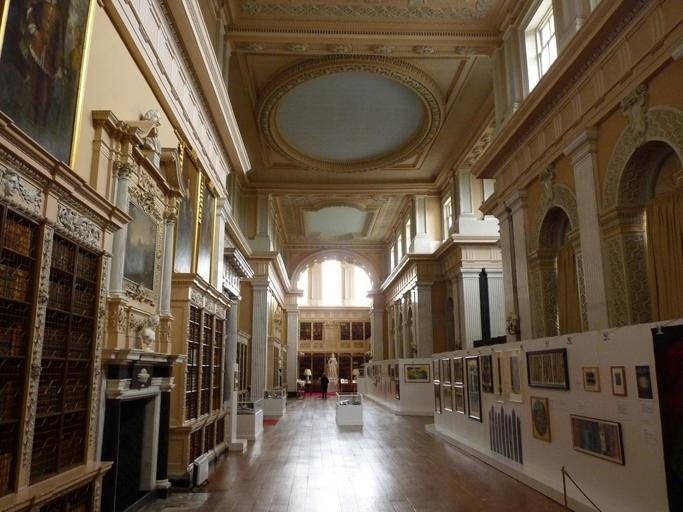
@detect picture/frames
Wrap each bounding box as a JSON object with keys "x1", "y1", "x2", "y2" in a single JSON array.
[{"x1": 373, "y1": 348, "x2": 653, "y2": 465}]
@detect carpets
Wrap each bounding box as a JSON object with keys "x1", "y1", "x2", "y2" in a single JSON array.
[{"x1": 263, "y1": 418, "x2": 279, "y2": 426}]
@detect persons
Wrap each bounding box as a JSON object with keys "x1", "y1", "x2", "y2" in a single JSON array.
[
  {"x1": 22, "y1": 0, "x2": 66, "y2": 131},
  {"x1": 319, "y1": 373, "x2": 329, "y2": 399},
  {"x1": 328, "y1": 352, "x2": 338, "y2": 379}
]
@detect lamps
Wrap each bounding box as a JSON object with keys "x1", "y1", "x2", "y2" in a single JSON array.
[
  {"x1": 352, "y1": 369, "x2": 361, "y2": 383},
  {"x1": 303, "y1": 369, "x2": 312, "y2": 384}
]
